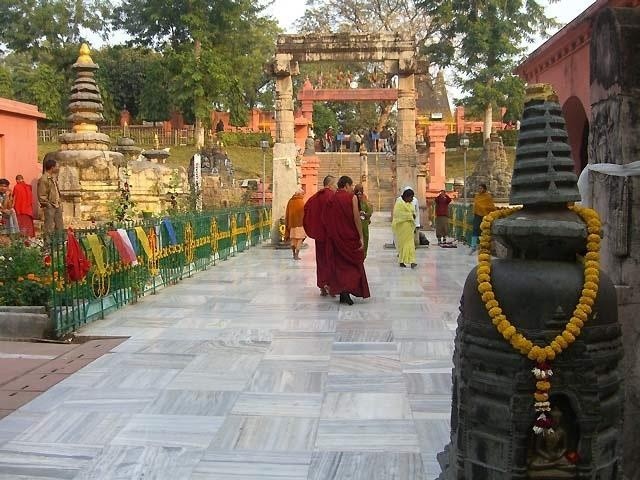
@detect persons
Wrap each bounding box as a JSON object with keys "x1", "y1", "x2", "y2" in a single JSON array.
[
  {"x1": 351, "y1": 184, "x2": 374, "y2": 261},
  {"x1": 529, "y1": 405, "x2": 577, "y2": 472},
  {"x1": 433, "y1": 190, "x2": 452, "y2": 243},
  {"x1": 396, "y1": 186, "x2": 420, "y2": 257},
  {"x1": 0, "y1": 179, "x2": 15, "y2": 237},
  {"x1": 391, "y1": 189, "x2": 418, "y2": 268},
  {"x1": 36, "y1": 158, "x2": 63, "y2": 234},
  {"x1": 468, "y1": 183, "x2": 496, "y2": 256},
  {"x1": 12, "y1": 175, "x2": 35, "y2": 238},
  {"x1": 320, "y1": 175, "x2": 371, "y2": 305},
  {"x1": 286, "y1": 187, "x2": 308, "y2": 260},
  {"x1": 304, "y1": 175, "x2": 338, "y2": 297},
  {"x1": 322, "y1": 126, "x2": 392, "y2": 152}
]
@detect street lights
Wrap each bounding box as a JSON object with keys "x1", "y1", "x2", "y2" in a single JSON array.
[
  {"x1": 257, "y1": 135, "x2": 270, "y2": 241},
  {"x1": 458, "y1": 130, "x2": 471, "y2": 244}
]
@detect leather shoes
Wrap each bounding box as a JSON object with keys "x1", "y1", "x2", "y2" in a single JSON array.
[
  {"x1": 400, "y1": 263, "x2": 407, "y2": 267},
  {"x1": 411, "y1": 263, "x2": 417, "y2": 269}
]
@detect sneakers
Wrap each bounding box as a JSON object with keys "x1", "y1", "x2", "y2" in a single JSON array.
[
  {"x1": 320, "y1": 284, "x2": 329, "y2": 296},
  {"x1": 339, "y1": 293, "x2": 354, "y2": 306}
]
